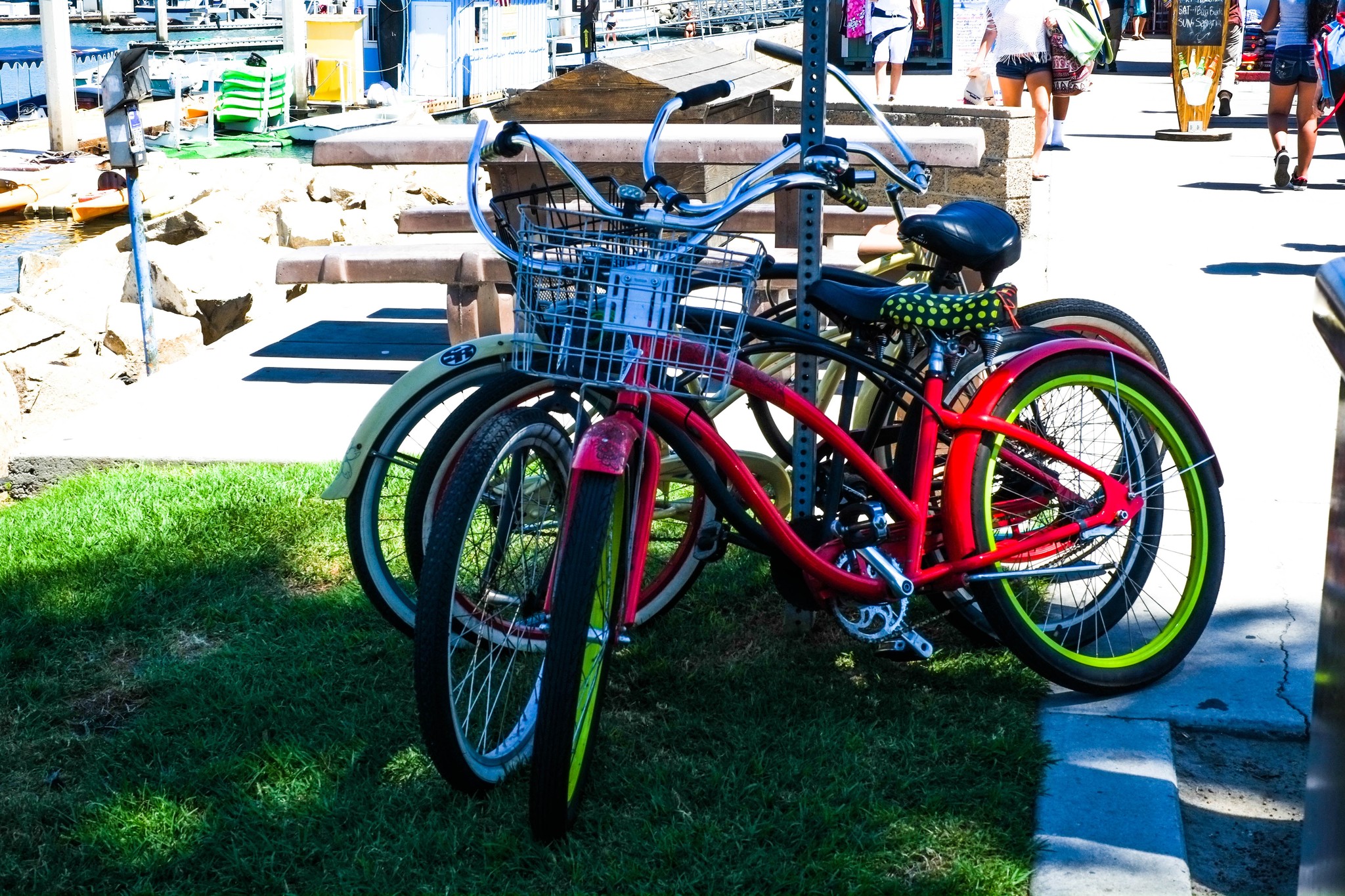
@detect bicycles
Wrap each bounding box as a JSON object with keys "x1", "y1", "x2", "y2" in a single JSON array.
[{"x1": 317, "y1": 37, "x2": 1226, "y2": 848}]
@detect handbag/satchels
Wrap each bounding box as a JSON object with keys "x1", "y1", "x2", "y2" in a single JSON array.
[
  {"x1": 1050, "y1": 27, "x2": 1093, "y2": 98},
  {"x1": 964, "y1": 74, "x2": 996, "y2": 106}
]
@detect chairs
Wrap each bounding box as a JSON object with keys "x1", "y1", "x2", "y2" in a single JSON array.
[{"x1": 0, "y1": 110, "x2": 10, "y2": 121}]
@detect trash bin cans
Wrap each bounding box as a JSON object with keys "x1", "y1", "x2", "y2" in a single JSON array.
[{"x1": 1293, "y1": 253, "x2": 1345, "y2": 892}]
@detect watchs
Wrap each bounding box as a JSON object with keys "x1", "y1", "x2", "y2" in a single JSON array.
[{"x1": 917, "y1": 12, "x2": 924, "y2": 18}]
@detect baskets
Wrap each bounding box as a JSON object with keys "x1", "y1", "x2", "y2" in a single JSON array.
[
  {"x1": 510, "y1": 204, "x2": 768, "y2": 401},
  {"x1": 490, "y1": 177, "x2": 709, "y2": 354}
]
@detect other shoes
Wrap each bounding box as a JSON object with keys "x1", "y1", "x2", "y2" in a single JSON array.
[
  {"x1": 1030, "y1": 170, "x2": 1045, "y2": 181},
  {"x1": 1139, "y1": 35, "x2": 1144, "y2": 40},
  {"x1": 1132, "y1": 35, "x2": 1141, "y2": 40},
  {"x1": 1218, "y1": 91, "x2": 1232, "y2": 117},
  {"x1": 1097, "y1": 64, "x2": 1105, "y2": 69},
  {"x1": 1108, "y1": 60, "x2": 1117, "y2": 72},
  {"x1": 888, "y1": 94, "x2": 894, "y2": 101}
]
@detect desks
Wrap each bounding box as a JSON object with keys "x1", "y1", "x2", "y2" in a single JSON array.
[
  {"x1": 313, "y1": 121, "x2": 987, "y2": 249},
  {"x1": 40, "y1": 104, "x2": 80, "y2": 107}
]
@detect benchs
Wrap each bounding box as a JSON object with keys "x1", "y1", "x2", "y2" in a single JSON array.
[
  {"x1": 398, "y1": 203, "x2": 941, "y2": 248},
  {"x1": 277, "y1": 249, "x2": 862, "y2": 346}
]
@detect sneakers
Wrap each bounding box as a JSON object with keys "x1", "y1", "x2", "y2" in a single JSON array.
[
  {"x1": 1290, "y1": 173, "x2": 1307, "y2": 190},
  {"x1": 1274, "y1": 145, "x2": 1290, "y2": 188}
]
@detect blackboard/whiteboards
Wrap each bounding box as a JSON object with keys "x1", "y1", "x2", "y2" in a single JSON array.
[{"x1": 1176, "y1": 0, "x2": 1225, "y2": 47}]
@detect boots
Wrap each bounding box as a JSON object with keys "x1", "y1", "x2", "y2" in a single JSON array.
[{"x1": 1052, "y1": 118, "x2": 1064, "y2": 147}]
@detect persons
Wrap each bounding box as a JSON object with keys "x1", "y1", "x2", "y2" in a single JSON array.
[
  {"x1": 871, "y1": 0, "x2": 924, "y2": 103},
  {"x1": 966, "y1": 0, "x2": 1154, "y2": 180},
  {"x1": 1209, "y1": 0, "x2": 1345, "y2": 192}
]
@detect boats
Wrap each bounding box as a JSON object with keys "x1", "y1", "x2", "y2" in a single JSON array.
[
  {"x1": 546, "y1": 0, "x2": 660, "y2": 40},
  {"x1": 0, "y1": 1, "x2": 288, "y2": 227},
  {"x1": 546, "y1": 35, "x2": 598, "y2": 77},
  {"x1": 651, "y1": 0, "x2": 804, "y2": 36}
]
